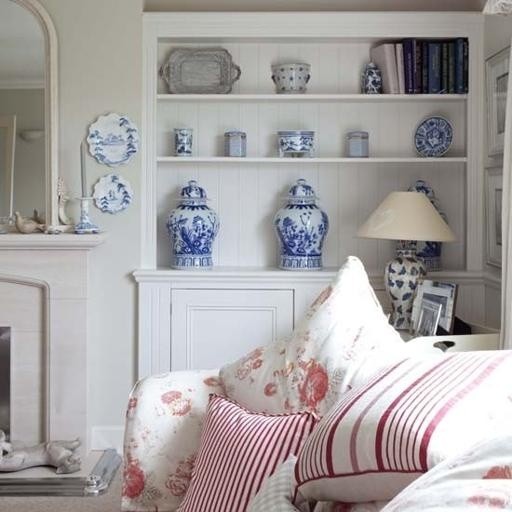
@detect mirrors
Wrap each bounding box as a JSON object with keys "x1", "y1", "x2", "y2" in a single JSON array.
[{"x1": 0, "y1": 0, "x2": 64, "y2": 233}]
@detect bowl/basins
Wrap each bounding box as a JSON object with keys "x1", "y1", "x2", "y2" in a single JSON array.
[{"x1": 277, "y1": 130, "x2": 314, "y2": 154}]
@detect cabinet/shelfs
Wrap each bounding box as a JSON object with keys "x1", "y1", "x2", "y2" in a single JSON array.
[
  {"x1": 133, "y1": 268, "x2": 490, "y2": 378},
  {"x1": 138, "y1": 9, "x2": 484, "y2": 279},
  {"x1": 478, "y1": 275, "x2": 504, "y2": 334}
]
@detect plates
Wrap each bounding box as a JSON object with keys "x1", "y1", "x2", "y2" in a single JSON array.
[
  {"x1": 93, "y1": 173, "x2": 134, "y2": 213},
  {"x1": 40, "y1": 224, "x2": 71, "y2": 234},
  {"x1": 86, "y1": 111, "x2": 138, "y2": 165},
  {"x1": 414, "y1": 116, "x2": 455, "y2": 157},
  {"x1": 164, "y1": 48, "x2": 233, "y2": 94}
]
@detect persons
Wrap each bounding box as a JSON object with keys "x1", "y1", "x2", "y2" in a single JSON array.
[{"x1": 419, "y1": 314, "x2": 434, "y2": 335}]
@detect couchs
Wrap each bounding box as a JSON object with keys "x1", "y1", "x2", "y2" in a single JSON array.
[{"x1": 117, "y1": 342, "x2": 510, "y2": 512}]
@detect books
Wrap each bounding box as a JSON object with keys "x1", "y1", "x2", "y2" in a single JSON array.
[{"x1": 371, "y1": 38, "x2": 468, "y2": 93}]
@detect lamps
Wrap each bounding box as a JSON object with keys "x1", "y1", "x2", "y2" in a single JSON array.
[{"x1": 351, "y1": 189, "x2": 460, "y2": 334}]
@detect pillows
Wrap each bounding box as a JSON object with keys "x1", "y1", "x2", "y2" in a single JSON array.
[
  {"x1": 292, "y1": 348, "x2": 511, "y2": 507},
  {"x1": 174, "y1": 393, "x2": 316, "y2": 512},
  {"x1": 214, "y1": 255, "x2": 404, "y2": 425},
  {"x1": 239, "y1": 454, "x2": 300, "y2": 512},
  {"x1": 382, "y1": 444, "x2": 510, "y2": 512}
]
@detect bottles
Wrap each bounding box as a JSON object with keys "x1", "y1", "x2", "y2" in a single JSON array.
[
  {"x1": 276, "y1": 178, "x2": 331, "y2": 272},
  {"x1": 361, "y1": 59, "x2": 383, "y2": 94},
  {"x1": 165, "y1": 178, "x2": 222, "y2": 272},
  {"x1": 405, "y1": 178, "x2": 451, "y2": 271}
]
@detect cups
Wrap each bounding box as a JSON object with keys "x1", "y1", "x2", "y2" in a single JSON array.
[
  {"x1": 174, "y1": 126, "x2": 192, "y2": 156},
  {"x1": 269, "y1": 61, "x2": 317, "y2": 94},
  {"x1": 346, "y1": 130, "x2": 370, "y2": 158},
  {"x1": 223, "y1": 132, "x2": 246, "y2": 158}
]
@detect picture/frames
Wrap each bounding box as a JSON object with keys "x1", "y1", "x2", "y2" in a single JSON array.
[
  {"x1": 413, "y1": 277, "x2": 460, "y2": 332},
  {"x1": 482, "y1": 45, "x2": 512, "y2": 158},
  {"x1": 413, "y1": 299, "x2": 443, "y2": 339},
  {"x1": 483, "y1": 164, "x2": 504, "y2": 272}
]
